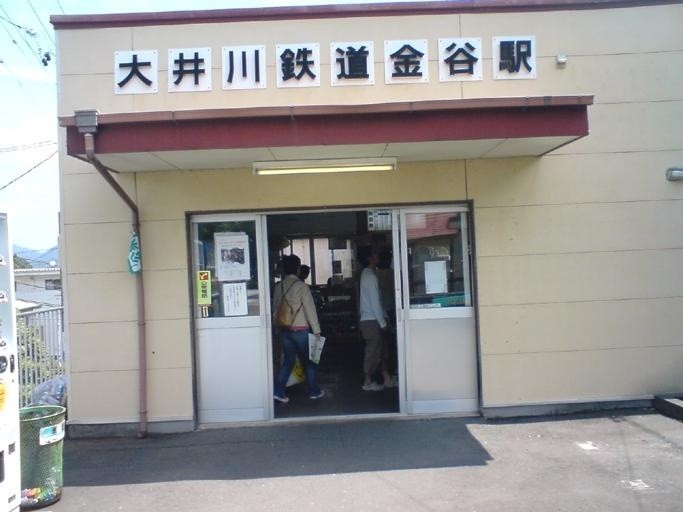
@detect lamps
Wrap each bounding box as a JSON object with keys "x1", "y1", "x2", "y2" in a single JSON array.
[{"x1": 252, "y1": 157, "x2": 398, "y2": 177}]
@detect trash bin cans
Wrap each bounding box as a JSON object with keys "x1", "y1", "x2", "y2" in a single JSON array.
[{"x1": 19, "y1": 405, "x2": 66, "y2": 511}]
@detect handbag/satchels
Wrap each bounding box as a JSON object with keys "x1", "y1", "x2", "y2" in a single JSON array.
[
  {"x1": 347, "y1": 321, "x2": 364, "y2": 348},
  {"x1": 280, "y1": 352, "x2": 305, "y2": 388}
]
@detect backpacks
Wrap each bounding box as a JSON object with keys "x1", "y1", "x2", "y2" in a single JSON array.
[{"x1": 272, "y1": 280, "x2": 303, "y2": 328}]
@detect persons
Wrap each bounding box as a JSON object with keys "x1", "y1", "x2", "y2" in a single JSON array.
[
  {"x1": 271, "y1": 254, "x2": 325, "y2": 402},
  {"x1": 375, "y1": 251, "x2": 398, "y2": 374},
  {"x1": 356, "y1": 245, "x2": 398, "y2": 391},
  {"x1": 299, "y1": 265, "x2": 310, "y2": 284}
]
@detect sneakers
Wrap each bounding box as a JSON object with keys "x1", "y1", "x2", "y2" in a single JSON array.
[
  {"x1": 309, "y1": 390, "x2": 324, "y2": 400},
  {"x1": 359, "y1": 382, "x2": 383, "y2": 391},
  {"x1": 273, "y1": 395, "x2": 288, "y2": 403},
  {"x1": 383, "y1": 380, "x2": 397, "y2": 388}
]
